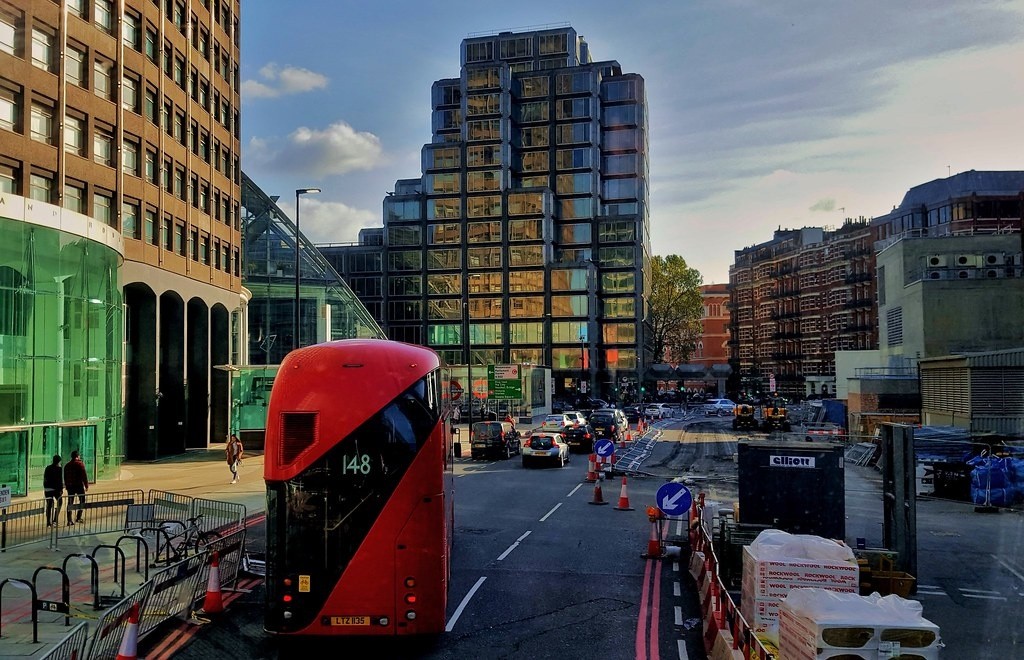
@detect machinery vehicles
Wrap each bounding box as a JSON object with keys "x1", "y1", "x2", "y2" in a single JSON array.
[{"x1": 733, "y1": 392, "x2": 791, "y2": 433}]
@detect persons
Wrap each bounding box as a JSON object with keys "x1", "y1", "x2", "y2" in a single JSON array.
[
  {"x1": 453, "y1": 406, "x2": 460, "y2": 424},
  {"x1": 64, "y1": 450, "x2": 88, "y2": 526},
  {"x1": 226, "y1": 434, "x2": 244, "y2": 484},
  {"x1": 43, "y1": 454, "x2": 63, "y2": 528},
  {"x1": 505, "y1": 414, "x2": 516, "y2": 430},
  {"x1": 739, "y1": 391, "x2": 793, "y2": 408},
  {"x1": 606, "y1": 387, "x2": 671, "y2": 407},
  {"x1": 480, "y1": 404, "x2": 485, "y2": 420}
]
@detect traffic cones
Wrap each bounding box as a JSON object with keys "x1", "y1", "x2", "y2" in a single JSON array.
[
  {"x1": 657, "y1": 412, "x2": 662, "y2": 422},
  {"x1": 587, "y1": 438, "x2": 616, "y2": 482},
  {"x1": 625, "y1": 425, "x2": 632, "y2": 441},
  {"x1": 614, "y1": 477, "x2": 635, "y2": 511},
  {"x1": 114, "y1": 602, "x2": 141, "y2": 660},
  {"x1": 588, "y1": 474, "x2": 609, "y2": 505},
  {"x1": 195, "y1": 550, "x2": 228, "y2": 618},
  {"x1": 640, "y1": 519, "x2": 663, "y2": 558},
  {"x1": 649, "y1": 414, "x2": 655, "y2": 423},
  {"x1": 638, "y1": 415, "x2": 648, "y2": 435},
  {"x1": 689, "y1": 500, "x2": 702, "y2": 547},
  {"x1": 619, "y1": 433, "x2": 626, "y2": 449}
]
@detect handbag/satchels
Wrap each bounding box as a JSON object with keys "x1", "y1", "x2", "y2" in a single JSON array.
[{"x1": 231, "y1": 460, "x2": 237, "y2": 472}]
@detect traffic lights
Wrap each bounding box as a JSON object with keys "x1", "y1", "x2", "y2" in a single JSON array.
[
  {"x1": 641, "y1": 381, "x2": 645, "y2": 391},
  {"x1": 677, "y1": 381, "x2": 682, "y2": 390},
  {"x1": 576, "y1": 379, "x2": 581, "y2": 391},
  {"x1": 614, "y1": 386, "x2": 618, "y2": 393}
]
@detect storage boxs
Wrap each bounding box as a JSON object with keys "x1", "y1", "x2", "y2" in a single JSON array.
[{"x1": 741, "y1": 545, "x2": 945, "y2": 660}]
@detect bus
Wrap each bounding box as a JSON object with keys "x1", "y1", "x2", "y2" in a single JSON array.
[{"x1": 261, "y1": 338, "x2": 450, "y2": 636}]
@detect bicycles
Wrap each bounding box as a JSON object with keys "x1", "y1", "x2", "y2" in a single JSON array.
[{"x1": 157, "y1": 513, "x2": 226, "y2": 565}]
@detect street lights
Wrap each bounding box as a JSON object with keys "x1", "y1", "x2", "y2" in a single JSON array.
[{"x1": 294, "y1": 188, "x2": 322, "y2": 351}]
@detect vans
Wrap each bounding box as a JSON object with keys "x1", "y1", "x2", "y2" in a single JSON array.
[{"x1": 470, "y1": 421, "x2": 522, "y2": 459}]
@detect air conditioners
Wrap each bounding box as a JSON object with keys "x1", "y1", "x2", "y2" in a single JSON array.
[{"x1": 927, "y1": 252, "x2": 1024, "y2": 279}]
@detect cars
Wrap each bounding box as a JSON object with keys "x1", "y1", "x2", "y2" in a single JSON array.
[
  {"x1": 542, "y1": 395, "x2": 630, "y2": 454},
  {"x1": 621, "y1": 403, "x2": 676, "y2": 424},
  {"x1": 522, "y1": 432, "x2": 571, "y2": 468},
  {"x1": 702, "y1": 398, "x2": 735, "y2": 417}
]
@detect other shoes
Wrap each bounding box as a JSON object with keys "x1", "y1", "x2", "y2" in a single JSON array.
[
  {"x1": 235, "y1": 475, "x2": 240, "y2": 483},
  {"x1": 46, "y1": 524, "x2": 54, "y2": 529},
  {"x1": 76, "y1": 519, "x2": 84, "y2": 523},
  {"x1": 230, "y1": 480, "x2": 237, "y2": 484},
  {"x1": 67, "y1": 522, "x2": 74, "y2": 526},
  {"x1": 53, "y1": 522, "x2": 60, "y2": 526}
]
